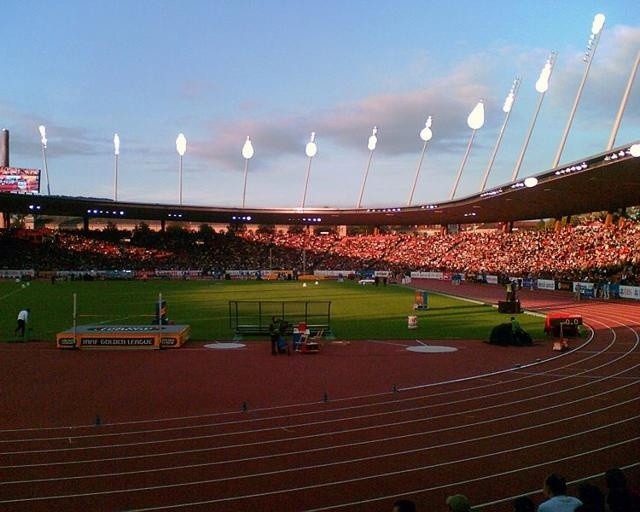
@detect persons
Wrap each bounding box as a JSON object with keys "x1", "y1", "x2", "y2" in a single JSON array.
[
  {"x1": 269, "y1": 316, "x2": 282, "y2": 356},
  {"x1": 390, "y1": 468, "x2": 640, "y2": 512},
  {"x1": 13, "y1": 308, "x2": 29, "y2": 336},
  {"x1": 511, "y1": 316, "x2": 521, "y2": 344},
  {"x1": 0, "y1": 208, "x2": 640, "y2": 298}
]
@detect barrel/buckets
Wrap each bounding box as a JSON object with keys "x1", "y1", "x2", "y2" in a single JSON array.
[
  {"x1": 415, "y1": 288, "x2": 428, "y2": 305},
  {"x1": 407, "y1": 315, "x2": 417, "y2": 330}
]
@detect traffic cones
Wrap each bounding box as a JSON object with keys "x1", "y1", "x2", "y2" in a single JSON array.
[
  {"x1": 241, "y1": 400, "x2": 247, "y2": 414},
  {"x1": 319, "y1": 387, "x2": 329, "y2": 404},
  {"x1": 95, "y1": 414, "x2": 101, "y2": 426},
  {"x1": 390, "y1": 383, "x2": 399, "y2": 392}
]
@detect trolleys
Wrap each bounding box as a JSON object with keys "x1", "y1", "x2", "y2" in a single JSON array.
[{"x1": 293, "y1": 327, "x2": 324, "y2": 354}]
